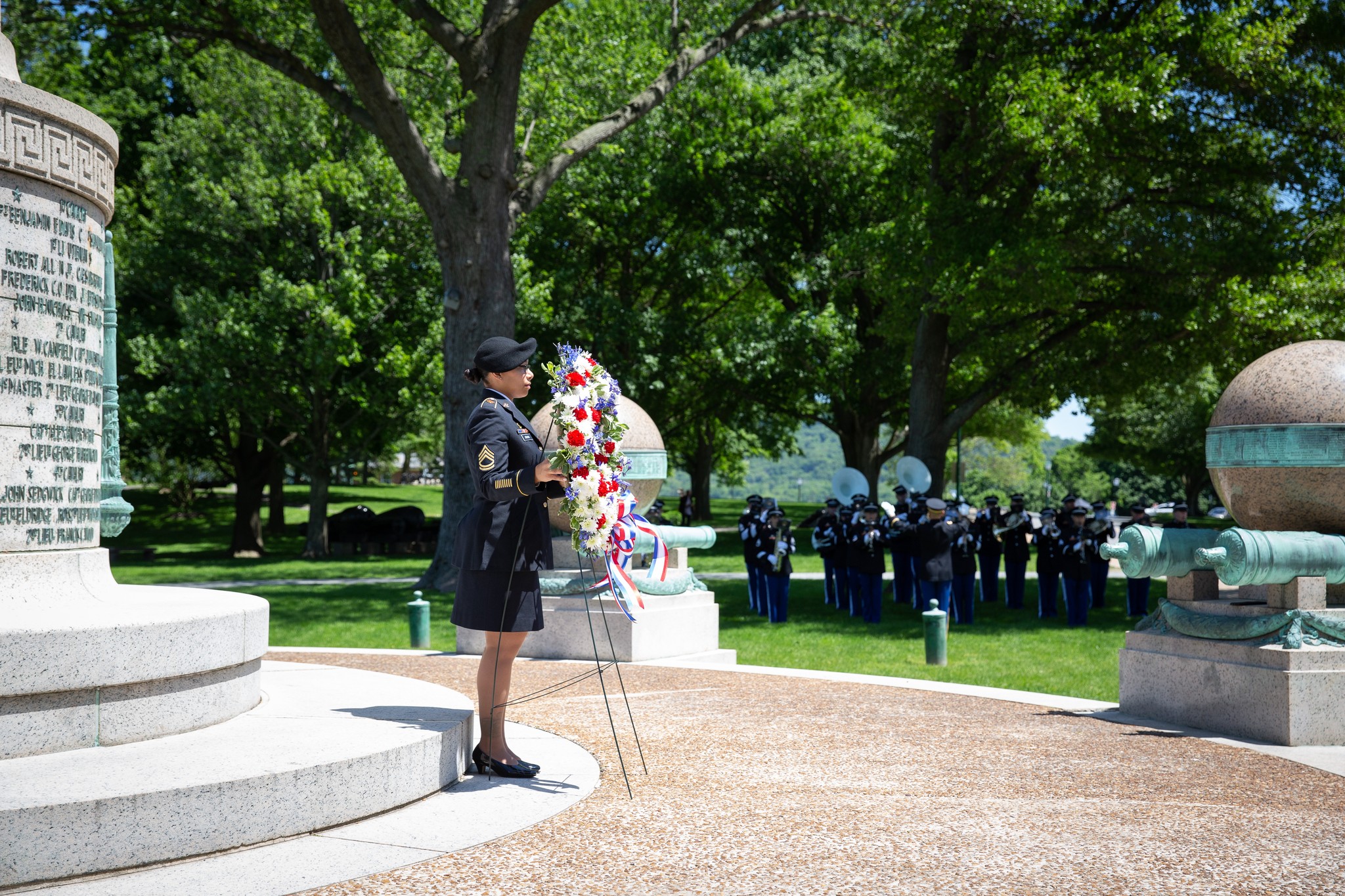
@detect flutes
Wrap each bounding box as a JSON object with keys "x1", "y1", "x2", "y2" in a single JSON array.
[
  {"x1": 1077, "y1": 525, "x2": 1089, "y2": 565},
  {"x1": 1044, "y1": 517, "x2": 1061, "y2": 538}
]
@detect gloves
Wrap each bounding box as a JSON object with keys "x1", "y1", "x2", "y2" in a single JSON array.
[
  {"x1": 767, "y1": 554, "x2": 778, "y2": 567},
  {"x1": 1041, "y1": 524, "x2": 1051, "y2": 536},
  {"x1": 777, "y1": 541, "x2": 789, "y2": 550},
  {"x1": 1020, "y1": 511, "x2": 1030, "y2": 523},
  {"x1": 958, "y1": 503, "x2": 970, "y2": 517},
  {"x1": 850, "y1": 511, "x2": 860, "y2": 524},
  {"x1": 880, "y1": 501, "x2": 895, "y2": 516},
  {"x1": 956, "y1": 534, "x2": 973, "y2": 548},
  {"x1": 863, "y1": 529, "x2": 880, "y2": 546},
  {"x1": 1072, "y1": 538, "x2": 1092, "y2": 553},
  {"x1": 759, "y1": 509, "x2": 768, "y2": 524}
]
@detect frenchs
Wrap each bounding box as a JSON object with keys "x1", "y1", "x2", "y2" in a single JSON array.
[
  {"x1": 992, "y1": 508, "x2": 1026, "y2": 542},
  {"x1": 896, "y1": 455, "x2": 932, "y2": 494},
  {"x1": 831, "y1": 467, "x2": 869, "y2": 507}
]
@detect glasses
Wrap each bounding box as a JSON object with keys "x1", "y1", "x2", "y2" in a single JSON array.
[{"x1": 519, "y1": 364, "x2": 530, "y2": 373}]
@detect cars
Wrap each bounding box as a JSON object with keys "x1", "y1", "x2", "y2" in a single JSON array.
[
  {"x1": 1145, "y1": 502, "x2": 1175, "y2": 518},
  {"x1": 1207, "y1": 507, "x2": 1232, "y2": 519}
]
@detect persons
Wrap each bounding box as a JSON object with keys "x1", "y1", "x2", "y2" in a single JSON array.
[
  {"x1": 461, "y1": 337, "x2": 572, "y2": 777},
  {"x1": 679, "y1": 490, "x2": 694, "y2": 527},
  {"x1": 738, "y1": 492, "x2": 1197, "y2": 631},
  {"x1": 644, "y1": 499, "x2": 672, "y2": 526}
]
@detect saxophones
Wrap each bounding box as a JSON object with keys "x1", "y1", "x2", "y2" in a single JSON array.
[{"x1": 771, "y1": 528, "x2": 783, "y2": 572}]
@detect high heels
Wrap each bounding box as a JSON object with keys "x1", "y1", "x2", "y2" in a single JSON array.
[{"x1": 472, "y1": 743, "x2": 540, "y2": 777}]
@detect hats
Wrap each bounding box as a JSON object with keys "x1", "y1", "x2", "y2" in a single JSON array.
[
  {"x1": 1091, "y1": 499, "x2": 1105, "y2": 510},
  {"x1": 825, "y1": 498, "x2": 840, "y2": 507},
  {"x1": 1071, "y1": 506, "x2": 1088, "y2": 516},
  {"x1": 1040, "y1": 507, "x2": 1057, "y2": 519},
  {"x1": 925, "y1": 498, "x2": 947, "y2": 514},
  {"x1": 1171, "y1": 499, "x2": 1189, "y2": 512},
  {"x1": 1130, "y1": 503, "x2": 1146, "y2": 514},
  {"x1": 473, "y1": 336, "x2": 537, "y2": 373},
  {"x1": 983, "y1": 496, "x2": 999, "y2": 505},
  {"x1": 916, "y1": 494, "x2": 969, "y2": 510},
  {"x1": 851, "y1": 494, "x2": 867, "y2": 503},
  {"x1": 863, "y1": 500, "x2": 881, "y2": 512},
  {"x1": 746, "y1": 495, "x2": 786, "y2": 518},
  {"x1": 1009, "y1": 493, "x2": 1024, "y2": 503},
  {"x1": 839, "y1": 506, "x2": 852, "y2": 515},
  {"x1": 1061, "y1": 493, "x2": 1077, "y2": 503},
  {"x1": 893, "y1": 485, "x2": 908, "y2": 496}
]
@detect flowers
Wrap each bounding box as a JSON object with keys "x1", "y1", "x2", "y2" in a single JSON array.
[{"x1": 542, "y1": 340, "x2": 633, "y2": 562}]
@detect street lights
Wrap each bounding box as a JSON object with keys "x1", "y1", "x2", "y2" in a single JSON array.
[
  {"x1": 796, "y1": 477, "x2": 803, "y2": 502},
  {"x1": 1112, "y1": 476, "x2": 1121, "y2": 502},
  {"x1": 1043, "y1": 459, "x2": 1052, "y2": 507}
]
